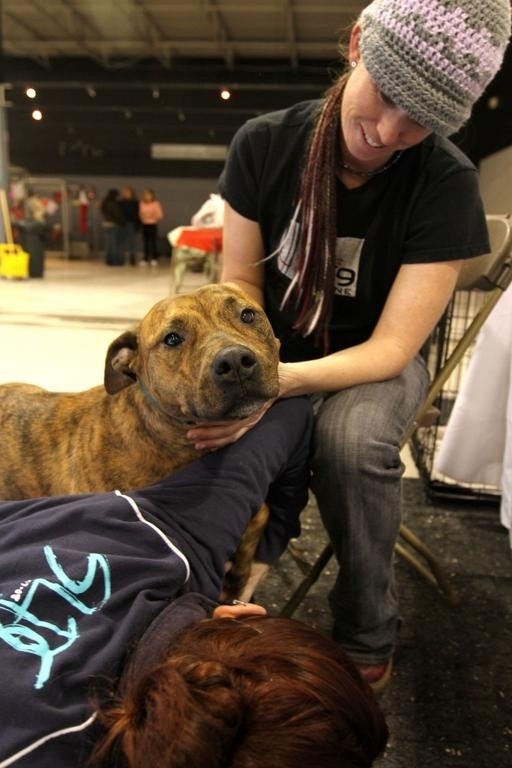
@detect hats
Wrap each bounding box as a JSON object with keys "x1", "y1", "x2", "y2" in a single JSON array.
[{"x1": 361, "y1": 0, "x2": 512, "y2": 140}]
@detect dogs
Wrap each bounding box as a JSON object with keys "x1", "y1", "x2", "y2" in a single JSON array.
[{"x1": 0, "y1": 281, "x2": 280, "y2": 504}]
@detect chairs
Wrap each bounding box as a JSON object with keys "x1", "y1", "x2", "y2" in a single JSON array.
[
  {"x1": 167, "y1": 226, "x2": 224, "y2": 293},
  {"x1": 265, "y1": 213, "x2": 512, "y2": 617}
]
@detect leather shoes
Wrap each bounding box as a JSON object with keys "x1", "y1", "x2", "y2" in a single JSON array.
[{"x1": 353, "y1": 656, "x2": 393, "y2": 694}]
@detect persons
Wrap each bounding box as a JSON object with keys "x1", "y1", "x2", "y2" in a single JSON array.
[
  {"x1": 99, "y1": 185, "x2": 165, "y2": 267},
  {"x1": 19, "y1": 196, "x2": 47, "y2": 278},
  {"x1": 187, "y1": 0, "x2": 512, "y2": 695},
  {"x1": 0, "y1": 397, "x2": 388, "y2": 768}
]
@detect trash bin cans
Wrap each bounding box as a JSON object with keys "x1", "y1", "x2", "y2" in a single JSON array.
[{"x1": 12, "y1": 221, "x2": 44, "y2": 277}]
